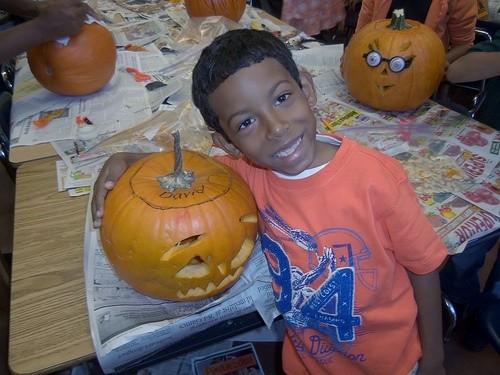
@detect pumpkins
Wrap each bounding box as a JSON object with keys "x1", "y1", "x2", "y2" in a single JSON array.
[
  {"x1": 184, "y1": 0, "x2": 247, "y2": 22},
  {"x1": 99, "y1": 150, "x2": 260, "y2": 301},
  {"x1": 27, "y1": 20, "x2": 118, "y2": 97},
  {"x1": 343, "y1": 18, "x2": 446, "y2": 112}
]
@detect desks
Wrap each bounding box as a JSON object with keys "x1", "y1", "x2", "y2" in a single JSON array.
[{"x1": 8, "y1": 10, "x2": 500, "y2": 375}]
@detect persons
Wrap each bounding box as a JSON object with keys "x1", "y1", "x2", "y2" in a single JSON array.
[
  {"x1": 246, "y1": 0, "x2": 477, "y2": 62},
  {"x1": 441, "y1": 39, "x2": 500, "y2": 354},
  {"x1": 90, "y1": 28, "x2": 449, "y2": 374},
  {"x1": 0, "y1": 0, "x2": 101, "y2": 131}
]
